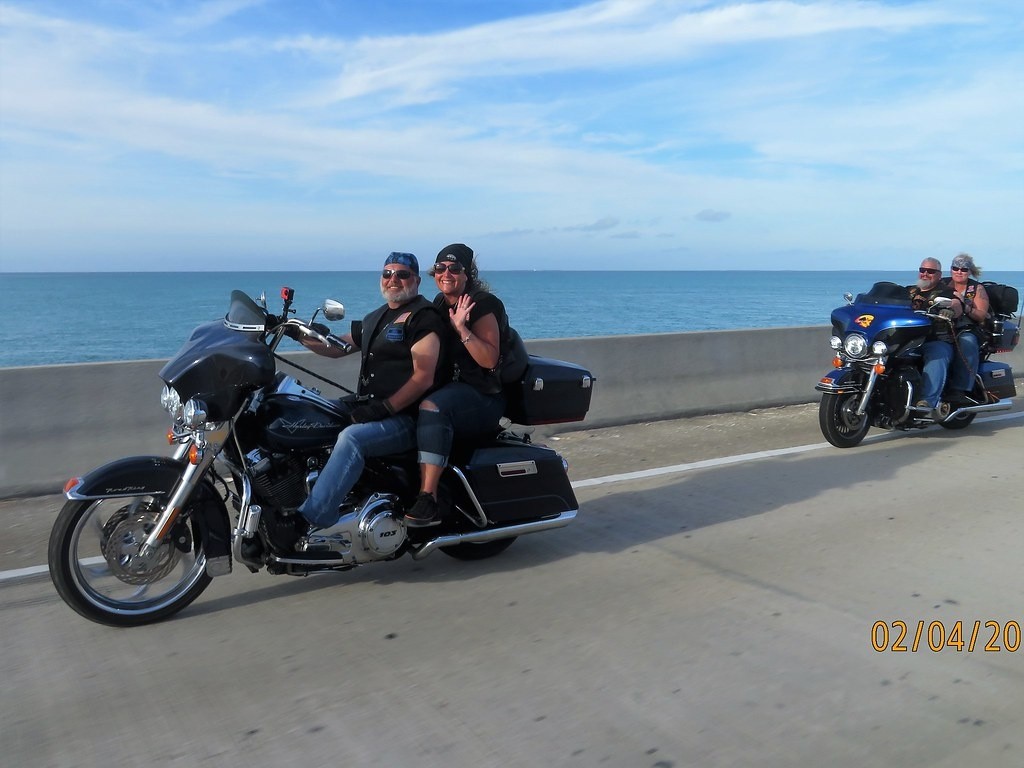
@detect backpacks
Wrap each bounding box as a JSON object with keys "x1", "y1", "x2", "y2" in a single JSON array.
[{"x1": 471, "y1": 288, "x2": 529, "y2": 385}]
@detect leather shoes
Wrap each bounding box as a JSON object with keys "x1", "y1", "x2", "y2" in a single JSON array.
[
  {"x1": 261, "y1": 511, "x2": 310, "y2": 555},
  {"x1": 404, "y1": 492, "x2": 442, "y2": 526}
]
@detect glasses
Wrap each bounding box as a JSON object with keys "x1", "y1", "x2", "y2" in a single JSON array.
[
  {"x1": 919, "y1": 267, "x2": 941, "y2": 274},
  {"x1": 383, "y1": 270, "x2": 418, "y2": 279},
  {"x1": 951, "y1": 266, "x2": 971, "y2": 272},
  {"x1": 434, "y1": 263, "x2": 465, "y2": 274}
]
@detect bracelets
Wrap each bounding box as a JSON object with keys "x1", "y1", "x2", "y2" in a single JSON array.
[
  {"x1": 458, "y1": 329, "x2": 473, "y2": 344},
  {"x1": 966, "y1": 304, "x2": 974, "y2": 317}
]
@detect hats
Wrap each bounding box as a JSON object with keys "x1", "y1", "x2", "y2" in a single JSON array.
[
  {"x1": 435, "y1": 244, "x2": 473, "y2": 273},
  {"x1": 384, "y1": 252, "x2": 419, "y2": 275}
]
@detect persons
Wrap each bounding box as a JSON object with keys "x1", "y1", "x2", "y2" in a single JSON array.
[
  {"x1": 266, "y1": 243, "x2": 509, "y2": 550},
  {"x1": 902, "y1": 254, "x2": 989, "y2": 412}
]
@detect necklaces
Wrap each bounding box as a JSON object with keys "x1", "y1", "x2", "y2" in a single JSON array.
[{"x1": 438, "y1": 296, "x2": 458, "y2": 316}]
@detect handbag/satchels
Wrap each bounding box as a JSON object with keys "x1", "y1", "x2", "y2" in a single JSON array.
[{"x1": 980, "y1": 281, "x2": 1019, "y2": 313}]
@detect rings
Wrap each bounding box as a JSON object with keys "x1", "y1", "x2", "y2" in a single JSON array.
[{"x1": 947, "y1": 315, "x2": 949, "y2": 317}]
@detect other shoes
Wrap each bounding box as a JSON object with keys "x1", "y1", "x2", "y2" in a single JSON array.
[{"x1": 916, "y1": 400, "x2": 928, "y2": 407}]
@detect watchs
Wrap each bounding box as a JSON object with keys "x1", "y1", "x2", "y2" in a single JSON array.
[{"x1": 946, "y1": 306, "x2": 954, "y2": 311}]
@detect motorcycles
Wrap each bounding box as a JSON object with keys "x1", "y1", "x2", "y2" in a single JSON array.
[
  {"x1": 815, "y1": 280, "x2": 1022, "y2": 448},
  {"x1": 46, "y1": 288, "x2": 596, "y2": 628}
]
